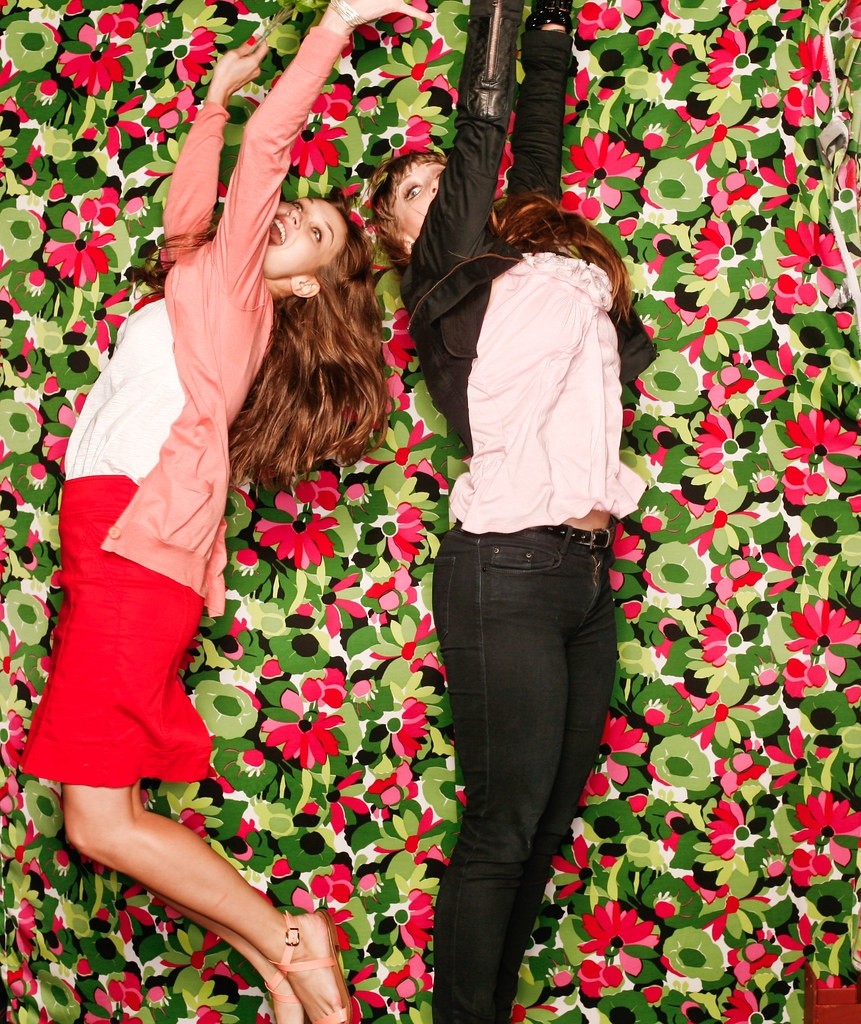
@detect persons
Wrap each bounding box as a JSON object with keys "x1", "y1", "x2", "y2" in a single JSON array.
[
  {"x1": 368, "y1": 0, "x2": 659, "y2": 1024},
  {"x1": 21, "y1": 0, "x2": 435, "y2": 1024}
]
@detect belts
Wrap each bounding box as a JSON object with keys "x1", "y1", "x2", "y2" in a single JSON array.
[{"x1": 527, "y1": 524, "x2": 614, "y2": 548}]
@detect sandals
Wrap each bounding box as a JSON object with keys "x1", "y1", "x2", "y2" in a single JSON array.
[
  {"x1": 264, "y1": 971, "x2": 304, "y2": 1024},
  {"x1": 268, "y1": 907, "x2": 351, "y2": 1024}
]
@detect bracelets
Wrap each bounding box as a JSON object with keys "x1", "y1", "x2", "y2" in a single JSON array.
[
  {"x1": 525, "y1": 9, "x2": 573, "y2": 34},
  {"x1": 329, "y1": 0, "x2": 367, "y2": 28}
]
